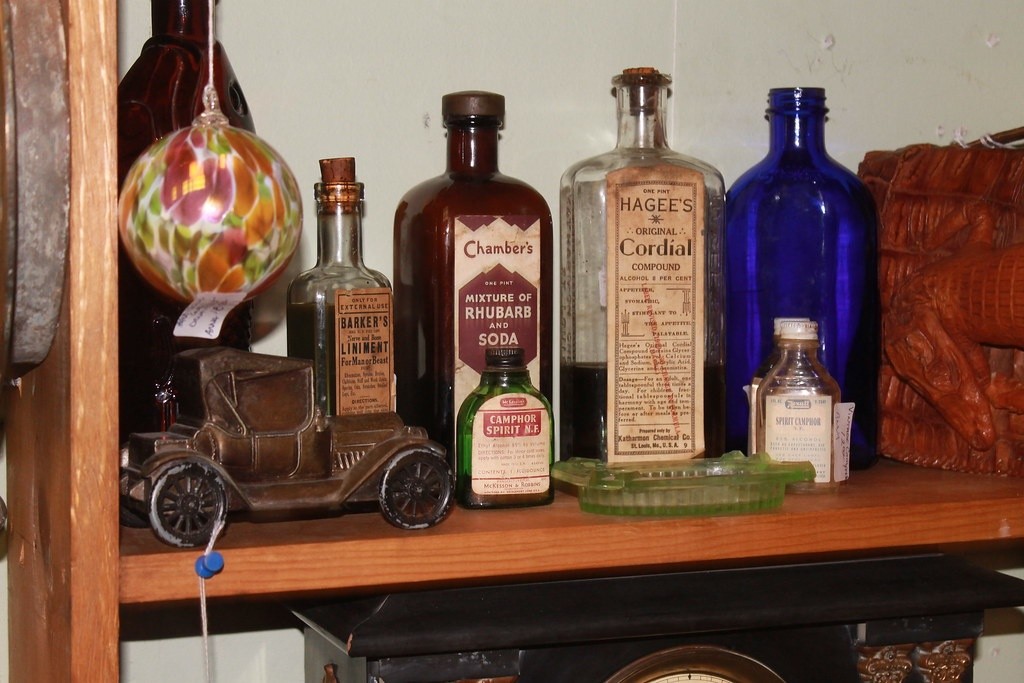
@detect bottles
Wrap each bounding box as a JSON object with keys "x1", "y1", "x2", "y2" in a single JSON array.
[
  {"x1": 766, "y1": 317, "x2": 848, "y2": 485},
  {"x1": 723, "y1": 87, "x2": 882, "y2": 471},
  {"x1": 756, "y1": 321, "x2": 839, "y2": 494},
  {"x1": 286, "y1": 156, "x2": 397, "y2": 418},
  {"x1": 557, "y1": 67, "x2": 728, "y2": 465},
  {"x1": 394, "y1": 90, "x2": 554, "y2": 494},
  {"x1": 456, "y1": 345, "x2": 555, "y2": 510}
]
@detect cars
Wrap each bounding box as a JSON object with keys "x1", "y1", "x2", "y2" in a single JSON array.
[{"x1": 120, "y1": 346, "x2": 457, "y2": 550}]
